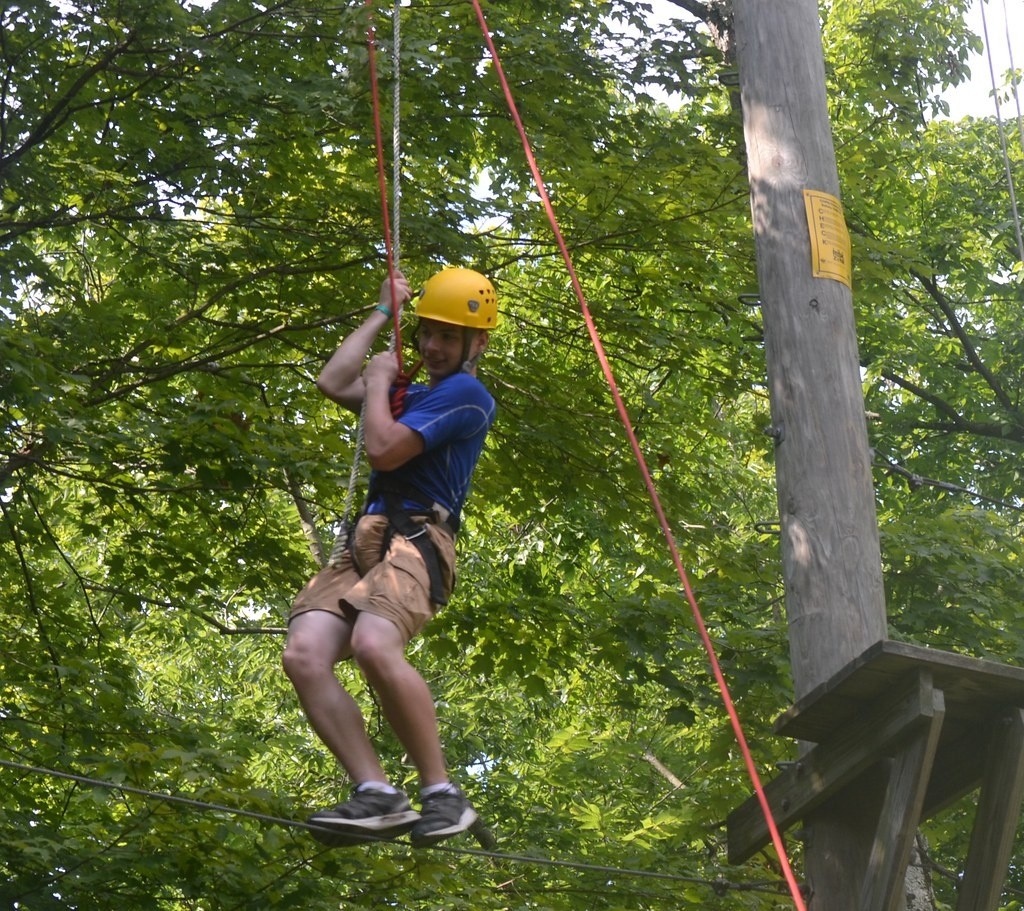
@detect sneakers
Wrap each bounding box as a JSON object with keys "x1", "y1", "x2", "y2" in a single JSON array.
[
  {"x1": 410, "y1": 781, "x2": 479, "y2": 849},
  {"x1": 307, "y1": 783, "x2": 422, "y2": 847}
]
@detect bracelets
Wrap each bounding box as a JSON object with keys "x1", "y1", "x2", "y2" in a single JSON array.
[{"x1": 373, "y1": 299, "x2": 391, "y2": 322}]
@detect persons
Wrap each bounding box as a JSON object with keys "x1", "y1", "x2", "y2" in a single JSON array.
[{"x1": 282, "y1": 263, "x2": 500, "y2": 845}]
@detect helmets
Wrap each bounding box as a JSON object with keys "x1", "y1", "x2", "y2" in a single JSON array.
[{"x1": 414, "y1": 268, "x2": 497, "y2": 329}]
